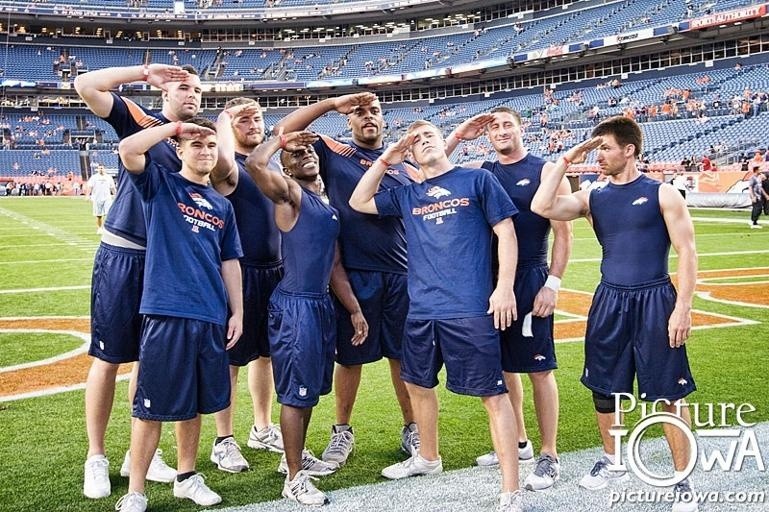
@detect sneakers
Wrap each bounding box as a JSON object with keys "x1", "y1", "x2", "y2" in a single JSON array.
[
  {"x1": 400, "y1": 422, "x2": 421, "y2": 456},
  {"x1": 475, "y1": 439, "x2": 534, "y2": 465},
  {"x1": 173, "y1": 472, "x2": 222, "y2": 507},
  {"x1": 210, "y1": 438, "x2": 249, "y2": 475},
  {"x1": 525, "y1": 454, "x2": 561, "y2": 492},
  {"x1": 381, "y1": 454, "x2": 443, "y2": 478},
  {"x1": 494, "y1": 489, "x2": 523, "y2": 512},
  {"x1": 670, "y1": 473, "x2": 698, "y2": 512},
  {"x1": 278, "y1": 424, "x2": 356, "y2": 508},
  {"x1": 82, "y1": 447, "x2": 178, "y2": 512},
  {"x1": 580, "y1": 452, "x2": 630, "y2": 490},
  {"x1": 248, "y1": 424, "x2": 286, "y2": 452}
]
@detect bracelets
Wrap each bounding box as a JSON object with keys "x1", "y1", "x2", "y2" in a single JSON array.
[
  {"x1": 143, "y1": 63, "x2": 150, "y2": 82},
  {"x1": 542, "y1": 275, "x2": 563, "y2": 293},
  {"x1": 378, "y1": 156, "x2": 392, "y2": 169},
  {"x1": 224, "y1": 110, "x2": 235, "y2": 119},
  {"x1": 563, "y1": 156, "x2": 572, "y2": 168},
  {"x1": 455, "y1": 130, "x2": 463, "y2": 142},
  {"x1": 175, "y1": 120, "x2": 183, "y2": 139},
  {"x1": 278, "y1": 135, "x2": 285, "y2": 150}
]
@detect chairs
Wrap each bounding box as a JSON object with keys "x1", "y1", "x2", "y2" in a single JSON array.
[{"x1": 0, "y1": 0, "x2": 769, "y2": 194}]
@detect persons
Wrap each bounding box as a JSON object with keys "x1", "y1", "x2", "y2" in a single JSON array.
[
  {"x1": 529, "y1": 117, "x2": 698, "y2": 512},
  {"x1": 444, "y1": 106, "x2": 575, "y2": 491},
  {"x1": 71, "y1": 63, "x2": 202, "y2": 500},
  {"x1": 0, "y1": 0, "x2": 768, "y2": 235},
  {"x1": 348, "y1": 121, "x2": 526, "y2": 512},
  {"x1": 274, "y1": 91, "x2": 421, "y2": 467},
  {"x1": 113, "y1": 119, "x2": 244, "y2": 512},
  {"x1": 205, "y1": 95, "x2": 284, "y2": 474},
  {"x1": 246, "y1": 129, "x2": 371, "y2": 507}
]
